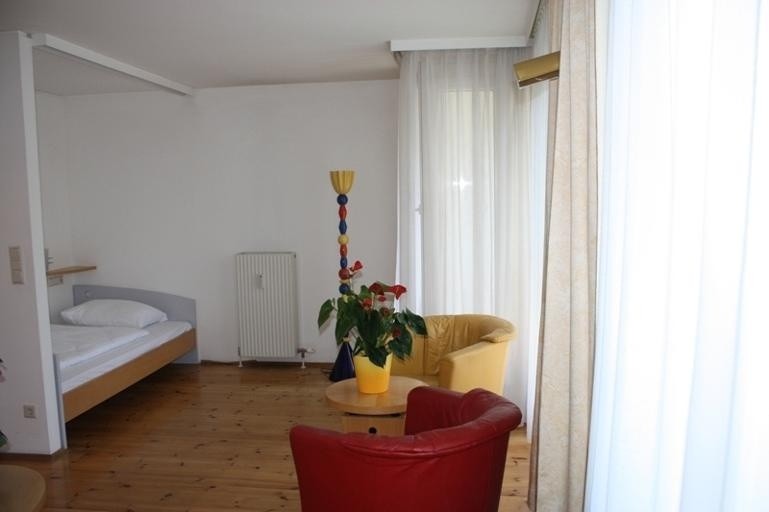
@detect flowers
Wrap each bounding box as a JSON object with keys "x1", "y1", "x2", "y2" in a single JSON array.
[{"x1": 316, "y1": 281, "x2": 425, "y2": 358}]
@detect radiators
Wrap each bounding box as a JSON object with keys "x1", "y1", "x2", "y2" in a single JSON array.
[{"x1": 235, "y1": 249, "x2": 316, "y2": 370}]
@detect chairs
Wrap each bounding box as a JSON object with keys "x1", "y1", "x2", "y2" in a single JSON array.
[
  {"x1": 392, "y1": 315, "x2": 517, "y2": 396},
  {"x1": 289, "y1": 387, "x2": 530, "y2": 512}
]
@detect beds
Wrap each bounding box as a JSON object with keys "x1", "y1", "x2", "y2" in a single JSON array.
[{"x1": 48, "y1": 282, "x2": 195, "y2": 426}]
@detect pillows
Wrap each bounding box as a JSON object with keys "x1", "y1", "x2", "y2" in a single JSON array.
[{"x1": 60, "y1": 297, "x2": 167, "y2": 328}]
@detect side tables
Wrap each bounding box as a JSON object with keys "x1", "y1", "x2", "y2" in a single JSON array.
[{"x1": 325, "y1": 376, "x2": 432, "y2": 437}]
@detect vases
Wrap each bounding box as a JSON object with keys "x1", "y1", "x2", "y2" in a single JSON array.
[{"x1": 352, "y1": 353, "x2": 392, "y2": 395}]
